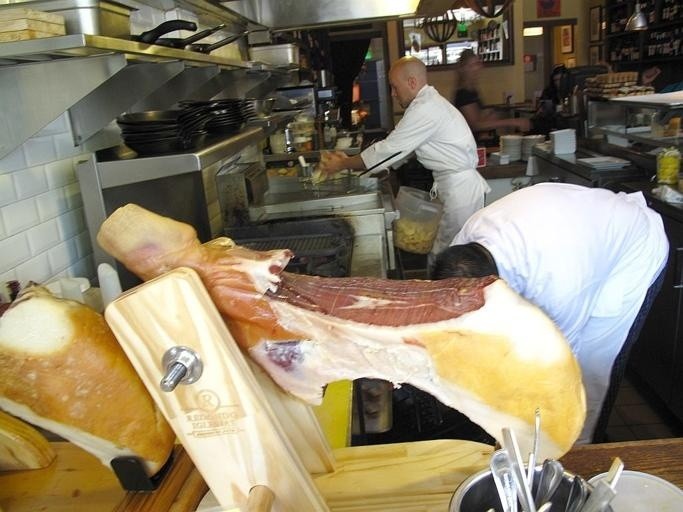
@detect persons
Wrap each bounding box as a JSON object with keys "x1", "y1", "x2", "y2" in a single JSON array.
[
  {"x1": 321, "y1": 54, "x2": 492, "y2": 280},
  {"x1": 536, "y1": 62, "x2": 571, "y2": 109},
  {"x1": 452, "y1": 48, "x2": 532, "y2": 147},
  {"x1": 430, "y1": 180, "x2": 671, "y2": 445}
]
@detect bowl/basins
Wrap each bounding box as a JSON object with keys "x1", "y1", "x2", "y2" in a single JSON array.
[
  {"x1": 448, "y1": 462, "x2": 613, "y2": 512},
  {"x1": 583, "y1": 470, "x2": 682, "y2": 512},
  {"x1": 254, "y1": 98, "x2": 276, "y2": 117}
]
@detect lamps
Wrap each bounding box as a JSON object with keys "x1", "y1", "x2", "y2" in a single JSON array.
[{"x1": 625, "y1": 0, "x2": 650, "y2": 31}]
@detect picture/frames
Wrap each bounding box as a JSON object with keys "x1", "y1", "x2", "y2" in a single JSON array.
[
  {"x1": 589, "y1": 3, "x2": 602, "y2": 66},
  {"x1": 559, "y1": 24, "x2": 575, "y2": 54}
]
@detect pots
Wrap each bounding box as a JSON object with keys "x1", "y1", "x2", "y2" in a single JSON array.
[
  {"x1": 129, "y1": 18, "x2": 249, "y2": 54},
  {"x1": 116, "y1": 96, "x2": 259, "y2": 158}
]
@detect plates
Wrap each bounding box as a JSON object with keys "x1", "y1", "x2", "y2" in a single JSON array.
[
  {"x1": 497, "y1": 127, "x2": 576, "y2": 162},
  {"x1": 575, "y1": 155, "x2": 632, "y2": 172}
]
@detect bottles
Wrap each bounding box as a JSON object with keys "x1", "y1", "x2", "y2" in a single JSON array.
[
  {"x1": 281, "y1": 129, "x2": 295, "y2": 166},
  {"x1": 639, "y1": 1, "x2": 683, "y2": 25},
  {"x1": 322, "y1": 124, "x2": 338, "y2": 142},
  {"x1": 525, "y1": 155, "x2": 539, "y2": 176},
  {"x1": 620, "y1": 28, "x2": 683, "y2": 61}
]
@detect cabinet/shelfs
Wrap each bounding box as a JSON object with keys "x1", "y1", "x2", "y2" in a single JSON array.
[
  {"x1": 478, "y1": 22, "x2": 503, "y2": 62},
  {"x1": 603, "y1": 0, "x2": 683, "y2": 65},
  {"x1": 586, "y1": 95, "x2": 682, "y2": 180}
]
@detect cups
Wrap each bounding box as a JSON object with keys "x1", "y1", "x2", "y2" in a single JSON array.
[{"x1": 654, "y1": 153, "x2": 680, "y2": 185}]
